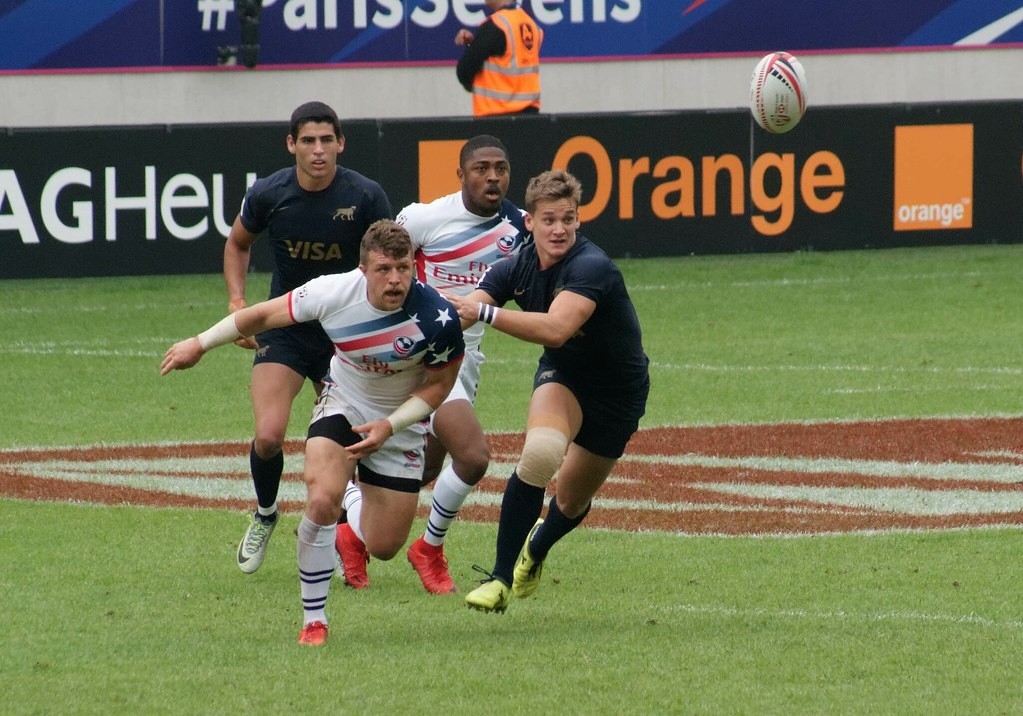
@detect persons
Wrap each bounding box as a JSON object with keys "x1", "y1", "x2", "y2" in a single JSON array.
[
  {"x1": 453, "y1": 0, "x2": 544, "y2": 117},
  {"x1": 160, "y1": 219, "x2": 465, "y2": 646},
  {"x1": 434, "y1": 169, "x2": 650, "y2": 613},
  {"x1": 335, "y1": 136, "x2": 535, "y2": 590},
  {"x1": 223, "y1": 101, "x2": 394, "y2": 579}
]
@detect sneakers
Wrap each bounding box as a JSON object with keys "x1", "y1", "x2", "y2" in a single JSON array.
[
  {"x1": 406, "y1": 533, "x2": 461, "y2": 595},
  {"x1": 298, "y1": 621, "x2": 330, "y2": 648},
  {"x1": 237, "y1": 508, "x2": 281, "y2": 574},
  {"x1": 512, "y1": 518, "x2": 545, "y2": 600},
  {"x1": 335, "y1": 522, "x2": 371, "y2": 590},
  {"x1": 464, "y1": 564, "x2": 511, "y2": 615}
]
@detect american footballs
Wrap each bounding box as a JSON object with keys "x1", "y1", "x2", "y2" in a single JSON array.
[{"x1": 748, "y1": 51, "x2": 810, "y2": 135}]
